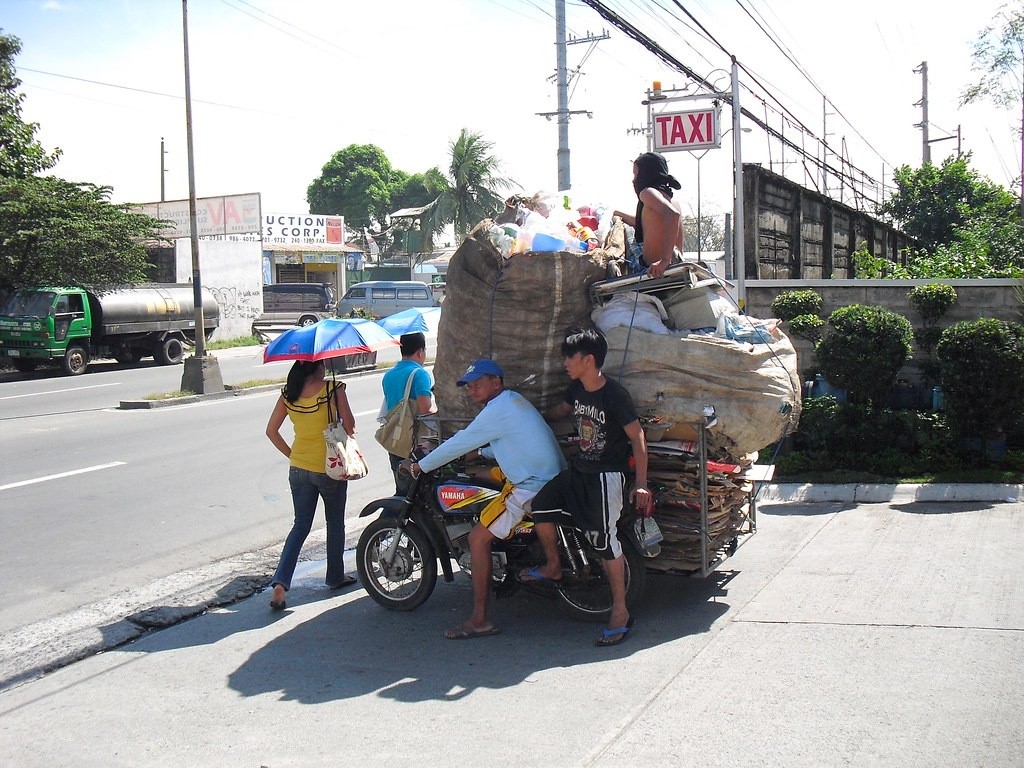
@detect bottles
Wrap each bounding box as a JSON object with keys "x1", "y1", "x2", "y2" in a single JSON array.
[{"x1": 489, "y1": 212, "x2": 586, "y2": 258}]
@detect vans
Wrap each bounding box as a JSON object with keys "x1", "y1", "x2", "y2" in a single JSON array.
[
  {"x1": 252, "y1": 282, "x2": 338, "y2": 327},
  {"x1": 335, "y1": 281, "x2": 434, "y2": 318}
]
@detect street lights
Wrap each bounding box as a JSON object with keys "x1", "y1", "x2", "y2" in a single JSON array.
[{"x1": 686, "y1": 127, "x2": 752, "y2": 262}]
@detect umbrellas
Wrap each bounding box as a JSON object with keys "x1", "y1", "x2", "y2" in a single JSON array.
[
  {"x1": 262, "y1": 318, "x2": 403, "y2": 381},
  {"x1": 376, "y1": 307, "x2": 441, "y2": 336}
]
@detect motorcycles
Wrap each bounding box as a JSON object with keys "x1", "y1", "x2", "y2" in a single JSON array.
[{"x1": 356, "y1": 412, "x2": 759, "y2": 624}]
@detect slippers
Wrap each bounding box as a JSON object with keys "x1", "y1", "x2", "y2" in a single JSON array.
[
  {"x1": 330, "y1": 575, "x2": 359, "y2": 591},
  {"x1": 442, "y1": 624, "x2": 501, "y2": 640},
  {"x1": 594, "y1": 615, "x2": 634, "y2": 644},
  {"x1": 269, "y1": 598, "x2": 289, "y2": 609},
  {"x1": 515, "y1": 564, "x2": 553, "y2": 583}
]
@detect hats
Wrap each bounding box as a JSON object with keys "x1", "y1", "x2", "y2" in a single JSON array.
[{"x1": 454, "y1": 357, "x2": 505, "y2": 386}]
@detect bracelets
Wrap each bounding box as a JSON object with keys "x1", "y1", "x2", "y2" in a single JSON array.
[
  {"x1": 410, "y1": 463, "x2": 421, "y2": 480},
  {"x1": 477, "y1": 448, "x2": 482, "y2": 459}
]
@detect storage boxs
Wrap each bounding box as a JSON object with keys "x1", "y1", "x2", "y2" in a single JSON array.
[{"x1": 668, "y1": 290, "x2": 737, "y2": 331}]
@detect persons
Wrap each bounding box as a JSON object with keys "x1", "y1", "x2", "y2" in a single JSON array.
[
  {"x1": 266, "y1": 359, "x2": 357, "y2": 610},
  {"x1": 382, "y1": 332, "x2": 432, "y2": 563},
  {"x1": 399, "y1": 358, "x2": 568, "y2": 639},
  {"x1": 514, "y1": 326, "x2": 650, "y2": 645},
  {"x1": 613, "y1": 151, "x2": 682, "y2": 278}
]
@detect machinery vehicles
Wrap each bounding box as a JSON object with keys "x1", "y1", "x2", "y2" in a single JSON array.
[{"x1": 0, "y1": 283, "x2": 220, "y2": 376}]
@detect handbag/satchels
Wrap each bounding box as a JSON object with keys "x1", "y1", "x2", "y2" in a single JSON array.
[
  {"x1": 373, "y1": 397, "x2": 436, "y2": 458},
  {"x1": 321, "y1": 421, "x2": 369, "y2": 480}
]
